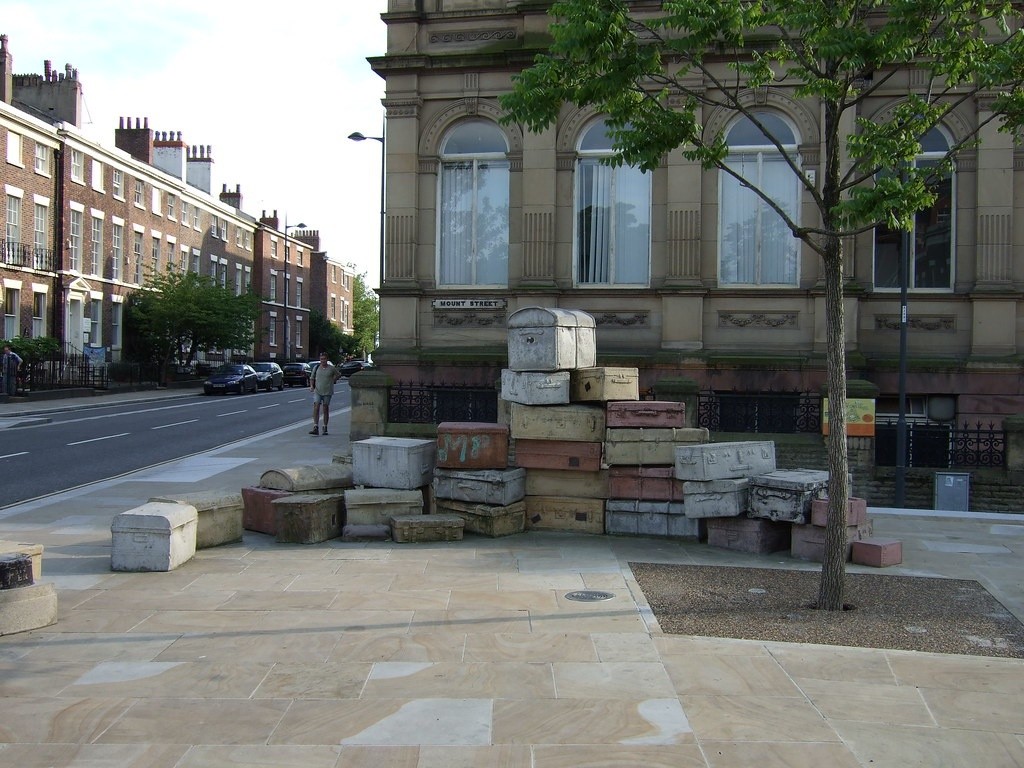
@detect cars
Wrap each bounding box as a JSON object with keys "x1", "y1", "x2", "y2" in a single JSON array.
[
  {"x1": 247, "y1": 361, "x2": 284, "y2": 392},
  {"x1": 307, "y1": 360, "x2": 338, "y2": 384},
  {"x1": 281, "y1": 362, "x2": 312, "y2": 387},
  {"x1": 340, "y1": 361, "x2": 372, "y2": 377},
  {"x1": 204, "y1": 364, "x2": 258, "y2": 396}
]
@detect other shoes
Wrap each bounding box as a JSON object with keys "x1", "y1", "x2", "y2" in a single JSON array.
[
  {"x1": 308, "y1": 426, "x2": 319, "y2": 435},
  {"x1": 322, "y1": 426, "x2": 328, "y2": 435}
]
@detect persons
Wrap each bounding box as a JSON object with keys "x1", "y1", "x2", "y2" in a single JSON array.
[
  {"x1": 1, "y1": 345, "x2": 23, "y2": 394},
  {"x1": 309, "y1": 353, "x2": 341, "y2": 435}
]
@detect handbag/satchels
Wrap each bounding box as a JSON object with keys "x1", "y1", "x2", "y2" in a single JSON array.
[{"x1": 313, "y1": 364, "x2": 320, "y2": 389}]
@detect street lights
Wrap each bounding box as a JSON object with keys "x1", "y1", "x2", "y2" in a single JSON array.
[{"x1": 346, "y1": 132, "x2": 385, "y2": 345}]
